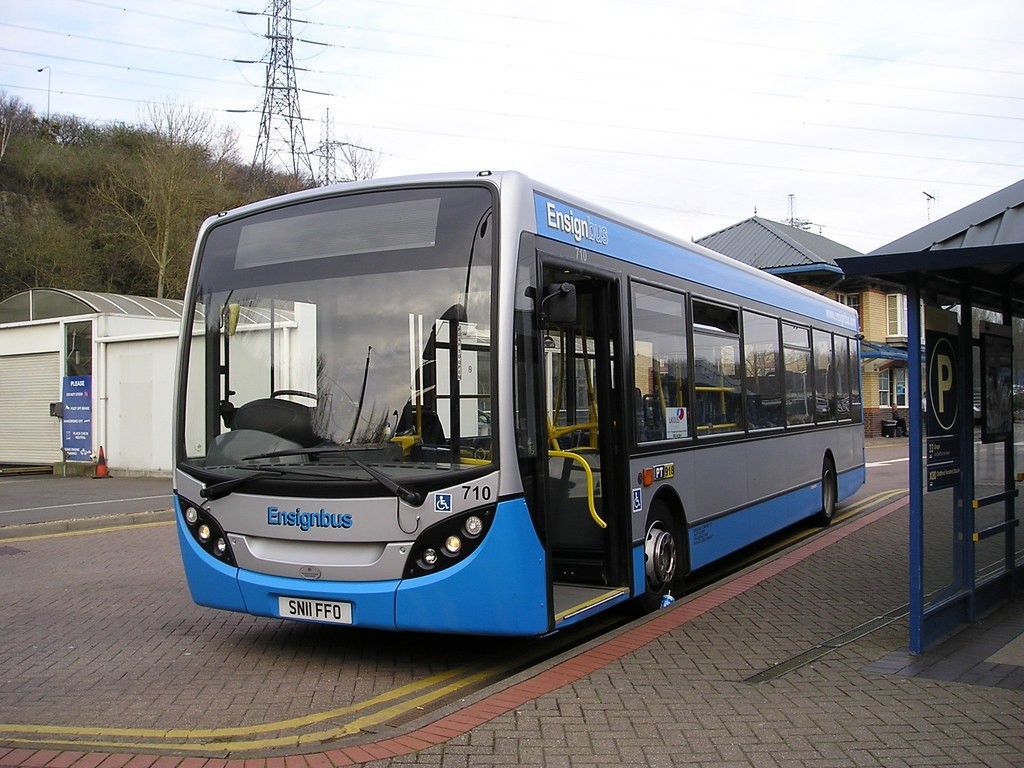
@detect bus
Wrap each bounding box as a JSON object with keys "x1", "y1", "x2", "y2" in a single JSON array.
[{"x1": 172, "y1": 169, "x2": 866, "y2": 638}]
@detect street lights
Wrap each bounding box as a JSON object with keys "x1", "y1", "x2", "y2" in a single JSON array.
[
  {"x1": 393, "y1": 410, "x2": 398, "y2": 430},
  {"x1": 464, "y1": 206, "x2": 492, "y2": 311}
]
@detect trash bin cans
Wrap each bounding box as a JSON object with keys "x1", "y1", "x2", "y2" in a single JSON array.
[{"x1": 882, "y1": 420, "x2": 898, "y2": 438}]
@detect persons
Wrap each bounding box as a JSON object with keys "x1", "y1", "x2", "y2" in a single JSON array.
[
  {"x1": 892, "y1": 403, "x2": 909, "y2": 437},
  {"x1": 921, "y1": 392, "x2": 928, "y2": 437}
]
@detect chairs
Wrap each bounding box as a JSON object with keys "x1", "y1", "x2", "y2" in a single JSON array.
[
  {"x1": 630, "y1": 387, "x2": 670, "y2": 444},
  {"x1": 407, "y1": 403, "x2": 446, "y2": 446}
]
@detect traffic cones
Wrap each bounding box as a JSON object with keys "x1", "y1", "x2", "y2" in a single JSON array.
[{"x1": 92, "y1": 446, "x2": 113, "y2": 479}]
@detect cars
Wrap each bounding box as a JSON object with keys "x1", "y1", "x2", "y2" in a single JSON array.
[
  {"x1": 477, "y1": 409, "x2": 491, "y2": 437},
  {"x1": 816, "y1": 398, "x2": 829, "y2": 416},
  {"x1": 987, "y1": 384, "x2": 1024, "y2": 407}
]
